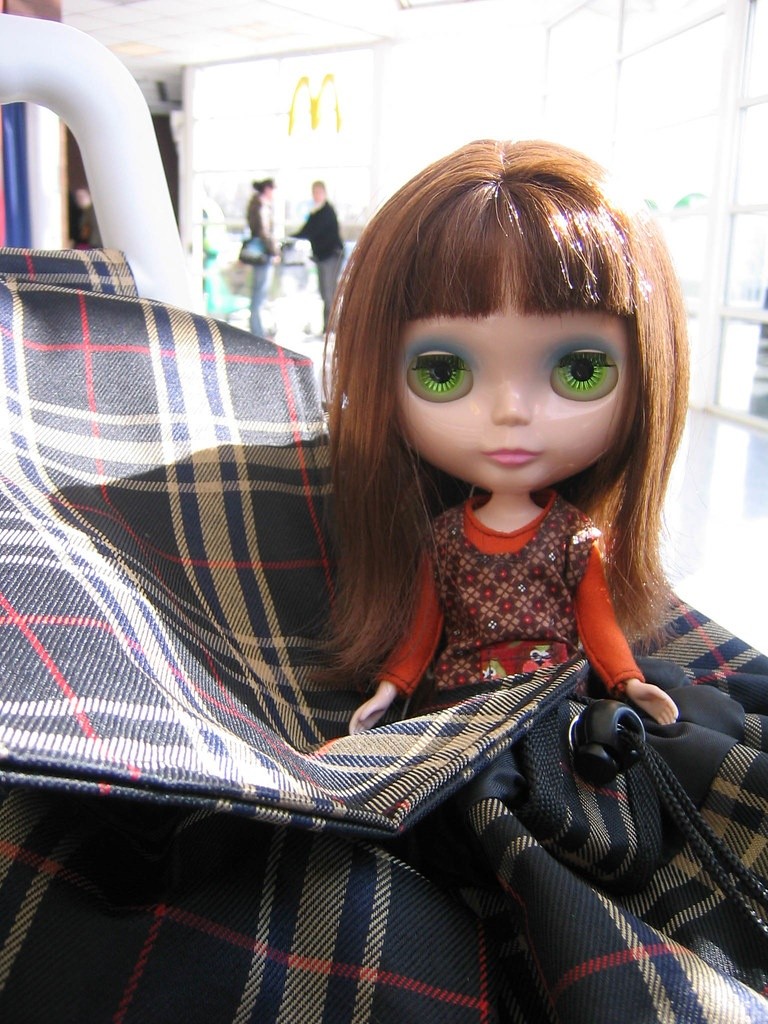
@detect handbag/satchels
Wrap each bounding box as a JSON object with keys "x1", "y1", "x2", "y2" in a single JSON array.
[{"x1": 239, "y1": 236, "x2": 270, "y2": 264}]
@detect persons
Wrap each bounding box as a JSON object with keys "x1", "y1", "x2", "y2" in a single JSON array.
[
  {"x1": 247, "y1": 179, "x2": 280, "y2": 339},
  {"x1": 313, "y1": 140, "x2": 689, "y2": 738},
  {"x1": 74, "y1": 190, "x2": 101, "y2": 250},
  {"x1": 295, "y1": 181, "x2": 344, "y2": 341}
]
